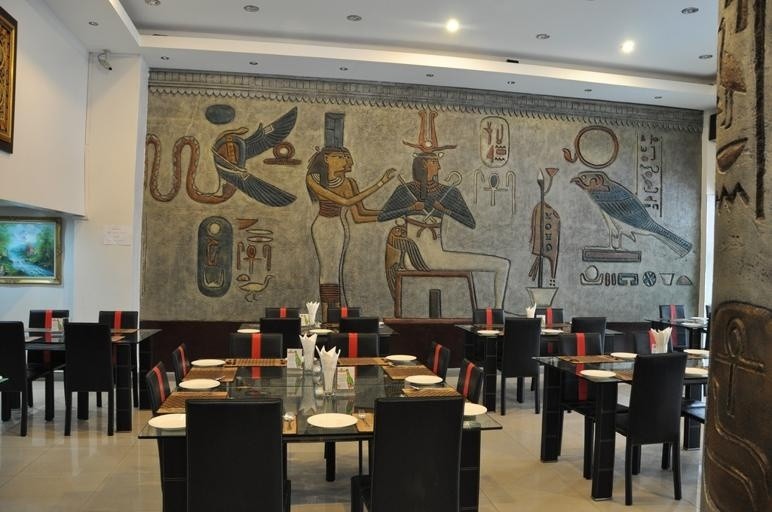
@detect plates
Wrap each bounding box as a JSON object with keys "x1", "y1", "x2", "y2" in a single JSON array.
[
  {"x1": 688, "y1": 317, "x2": 710, "y2": 321},
  {"x1": 177, "y1": 379, "x2": 221, "y2": 391},
  {"x1": 477, "y1": 330, "x2": 500, "y2": 335},
  {"x1": 237, "y1": 329, "x2": 260, "y2": 333},
  {"x1": 405, "y1": 373, "x2": 443, "y2": 387},
  {"x1": 541, "y1": 330, "x2": 564, "y2": 335},
  {"x1": 580, "y1": 369, "x2": 616, "y2": 378},
  {"x1": 149, "y1": 412, "x2": 185, "y2": 430},
  {"x1": 681, "y1": 322, "x2": 702, "y2": 328},
  {"x1": 191, "y1": 357, "x2": 227, "y2": 366},
  {"x1": 308, "y1": 328, "x2": 333, "y2": 334},
  {"x1": 308, "y1": 413, "x2": 359, "y2": 428},
  {"x1": 378, "y1": 321, "x2": 385, "y2": 325},
  {"x1": 610, "y1": 352, "x2": 638, "y2": 359},
  {"x1": 684, "y1": 367, "x2": 708, "y2": 376},
  {"x1": 683, "y1": 349, "x2": 710, "y2": 355},
  {"x1": 386, "y1": 353, "x2": 417, "y2": 363}
]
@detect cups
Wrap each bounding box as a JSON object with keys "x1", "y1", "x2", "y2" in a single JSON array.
[
  {"x1": 302, "y1": 342, "x2": 316, "y2": 371},
  {"x1": 308, "y1": 308, "x2": 316, "y2": 326}
]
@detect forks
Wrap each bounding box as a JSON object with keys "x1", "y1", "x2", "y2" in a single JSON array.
[{"x1": 356, "y1": 409, "x2": 372, "y2": 429}]
